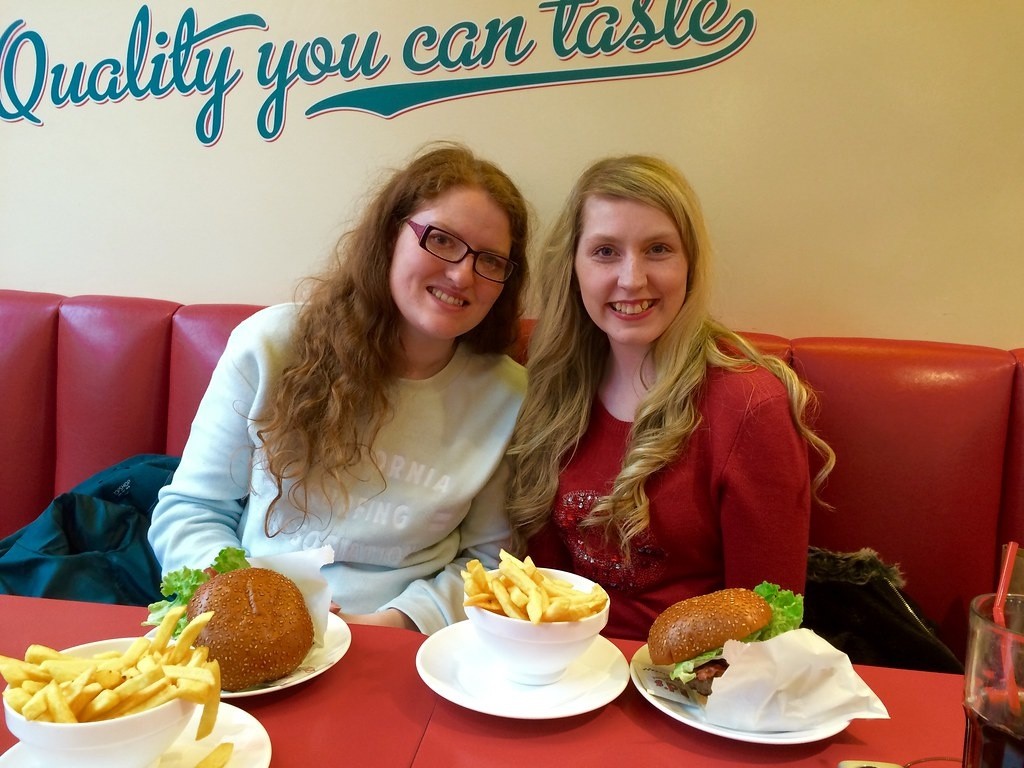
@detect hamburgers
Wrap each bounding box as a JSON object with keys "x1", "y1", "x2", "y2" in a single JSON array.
[
  {"x1": 648, "y1": 580, "x2": 804, "y2": 706},
  {"x1": 139, "y1": 546, "x2": 314, "y2": 691}
]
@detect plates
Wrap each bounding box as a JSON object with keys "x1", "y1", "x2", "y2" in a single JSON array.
[
  {"x1": 415, "y1": 621, "x2": 630, "y2": 719},
  {"x1": 630, "y1": 644, "x2": 852, "y2": 744},
  {"x1": 0, "y1": 701, "x2": 272, "y2": 768},
  {"x1": 142, "y1": 612, "x2": 351, "y2": 697}
]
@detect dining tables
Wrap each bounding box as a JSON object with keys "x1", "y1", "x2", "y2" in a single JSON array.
[{"x1": 0, "y1": 592, "x2": 966, "y2": 768}]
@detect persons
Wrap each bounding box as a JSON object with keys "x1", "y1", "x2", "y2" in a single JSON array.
[
  {"x1": 148, "y1": 146, "x2": 529, "y2": 635},
  {"x1": 508, "y1": 156, "x2": 810, "y2": 641}
]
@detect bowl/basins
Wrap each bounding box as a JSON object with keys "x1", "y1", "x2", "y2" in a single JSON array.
[
  {"x1": 3, "y1": 637, "x2": 207, "y2": 768},
  {"x1": 463, "y1": 567, "x2": 610, "y2": 685}
]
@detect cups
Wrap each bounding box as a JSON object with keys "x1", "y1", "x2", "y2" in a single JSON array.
[{"x1": 961, "y1": 592, "x2": 1024, "y2": 768}]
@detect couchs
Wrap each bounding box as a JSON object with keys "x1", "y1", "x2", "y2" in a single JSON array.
[{"x1": 0, "y1": 289, "x2": 1023, "y2": 670}]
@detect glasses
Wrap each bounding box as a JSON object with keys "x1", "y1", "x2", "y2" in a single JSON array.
[{"x1": 405, "y1": 218, "x2": 519, "y2": 283}]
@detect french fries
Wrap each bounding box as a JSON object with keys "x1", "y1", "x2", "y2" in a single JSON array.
[
  {"x1": 459, "y1": 547, "x2": 608, "y2": 626},
  {"x1": 0, "y1": 604, "x2": 234, "y2": 767}
]
